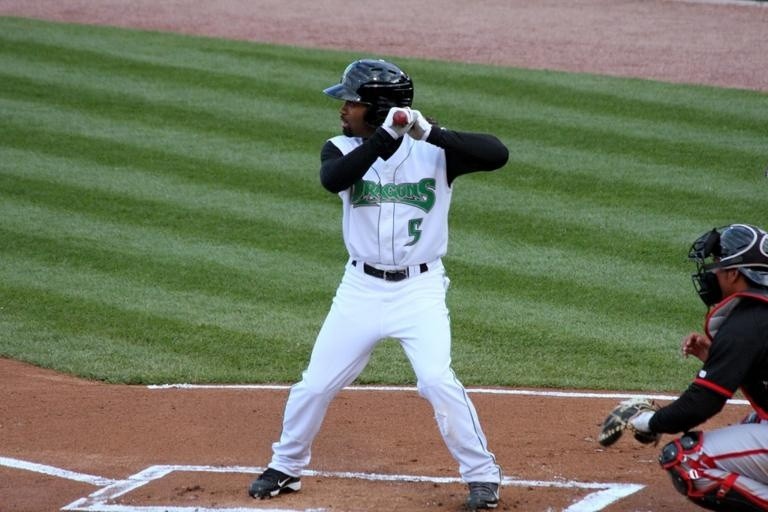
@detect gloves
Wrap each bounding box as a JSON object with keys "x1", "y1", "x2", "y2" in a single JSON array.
[{"x1": 380, "y1": 105, "x2": 432, "y2": 142}]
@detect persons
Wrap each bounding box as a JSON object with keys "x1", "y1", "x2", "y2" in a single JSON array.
[
  {"x1": 588, "y1": 221, "x2": 768, "y2": 512},
  {"x1": 245, "y1": 57, "x2": 513, "y2": 512}
]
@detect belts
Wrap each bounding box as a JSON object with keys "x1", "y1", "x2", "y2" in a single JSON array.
[{"x1": 351, "y1": 259, "x2": 427, "y2": 282}]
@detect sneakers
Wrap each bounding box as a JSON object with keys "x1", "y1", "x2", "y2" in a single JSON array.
[
  {"x1": 467, "y1": 481, "x2": 500, "y2": 508},
  {"x1": 249, "y1": 468, "x2": 301, "y2": 500}
]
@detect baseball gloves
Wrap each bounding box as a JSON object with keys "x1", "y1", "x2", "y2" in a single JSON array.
[{"x1": 598, "y1": 398, "x2": 666, "y2": 447}]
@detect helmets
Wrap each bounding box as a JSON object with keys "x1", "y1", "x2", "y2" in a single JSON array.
[
  {"x1": 688, "y1": 223, "x2": 768, "y2": 307},
  {"x1": 323, "y1": 59, "x2": 414, "y2": 109}
]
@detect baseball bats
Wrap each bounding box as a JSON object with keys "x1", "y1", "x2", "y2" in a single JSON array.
[{"x1": 393, "y1": 112, "x2": 438, "y2": 126}]
